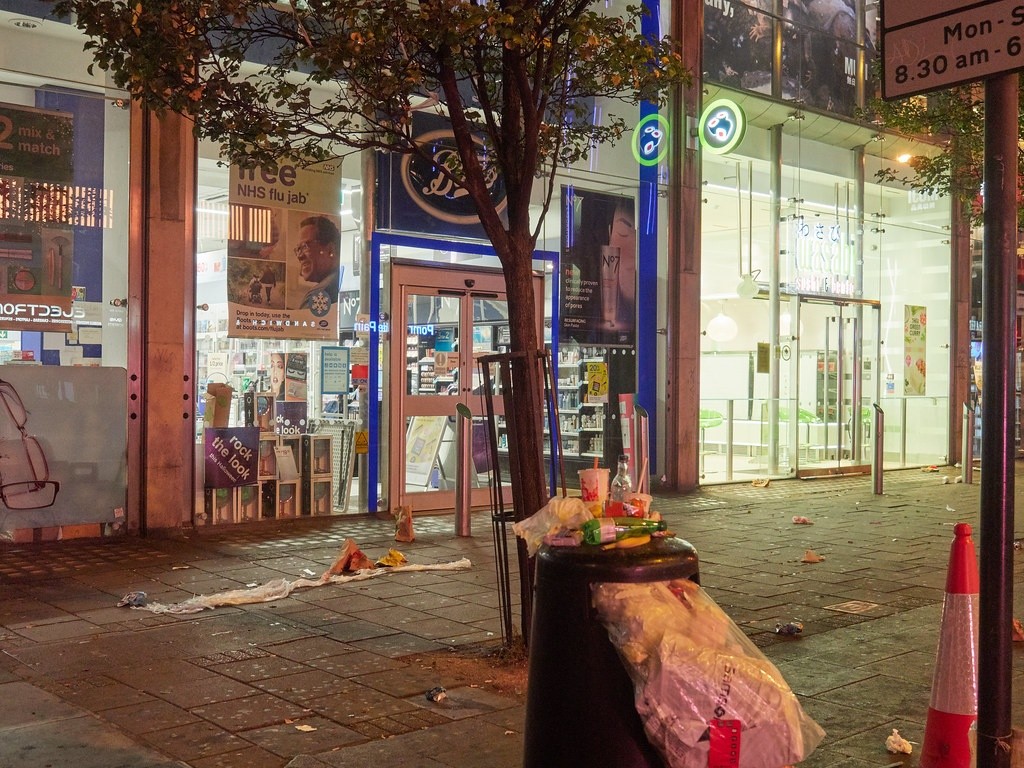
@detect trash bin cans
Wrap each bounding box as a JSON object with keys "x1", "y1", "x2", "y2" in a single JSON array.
[{"x1": 518, "y1": 524, "x2": 704, "y2": 768}]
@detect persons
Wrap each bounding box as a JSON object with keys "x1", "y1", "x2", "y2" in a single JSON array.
[
  {"x1": 293, "y1": 217, "x2": 341, "y2": 309},
  {"x1": 260, "y1": 265, "x2": 277, "y2": 305},
  {"x1": 269, "y1": 352, "x2": 286, "y2": 401},
  {"x1": 248, "y1": 272, "x2": 262, "y2": 301}
]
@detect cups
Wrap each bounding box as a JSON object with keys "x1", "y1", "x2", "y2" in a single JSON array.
[
  {"x1": 623, "y1": 494, "x2": 653, "y2": 518},
  {"x1": 577, "y1": 468, "x2": 610, "y2": 518}
]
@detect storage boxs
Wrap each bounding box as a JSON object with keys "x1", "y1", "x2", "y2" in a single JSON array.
[{"x1": 200, "y1": 382, "x2": 334, "y2": 526}]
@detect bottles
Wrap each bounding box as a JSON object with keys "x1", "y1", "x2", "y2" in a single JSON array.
[
  {"x1": 601, "y1": 533, "x2": 651, "y2": 551},
  {"x1": 582, "y1": 517, "x2": 668, "y2": 545},
  {"x1": 611, "y1": 455, "x2": 632, "y2": 501}
]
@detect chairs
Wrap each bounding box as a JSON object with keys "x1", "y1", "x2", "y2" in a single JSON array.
[
  {"x1": 847, "y1": 405, "x2": 871, "y2": 461},
  {"x1": 795, "y1": 410, "x2": 821, "y2": 467},
  {"x1": 698, "y1": 410, "x2": 725, "y2": 478}
]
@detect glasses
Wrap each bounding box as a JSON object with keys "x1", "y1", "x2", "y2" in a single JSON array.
[
  {"x1": 0, "y1": 378, "x2": 49, "y2": 499},
  {"x1": 293, "y1": 240, "x2": 320, "y2": 257},
  {"x1": 0, "y1": 481, "x2": 60, "y2": 510}
]
